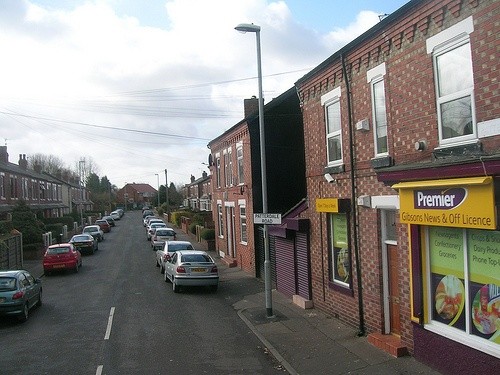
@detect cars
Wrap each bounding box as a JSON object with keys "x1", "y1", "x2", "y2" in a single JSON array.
[
  {"x1": 102, "y1": 208, "x2": 124, "y2": 227},
  {"x1": 43, "y1": 242, "x2": 82, "y2": 277},
  {"x1": 163, "y1": 249, "x2": 220, "y2": 293},
  {"x1": 95, "y1": 219, "x2": 111, "y2": 233},
  {"x1": 0, "y1": 269, "x2": 43, "y2": 322},
  {"x1": 81, "y1": 224, "x2": 105, "y2": 242},
  {"x1": 68, "y1": 234, "x2": 99, "y2": 255},
  {"x1": 141, "y1": 206, "x2": 176, "y2": 251},
  {"x1": 155, "y1": 240, "x2": 196, "y2": 275}
]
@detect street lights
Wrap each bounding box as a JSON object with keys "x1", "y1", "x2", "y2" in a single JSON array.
[
  {"x1": 78, "y1": 161, "x2": 85, "y2": 229},
  {"x1": 155, "y1": 173, "x2": 160, "y2": 206},
  {"x1": 234, "y1": 22, "x2": 273, "y2": 317}
]
